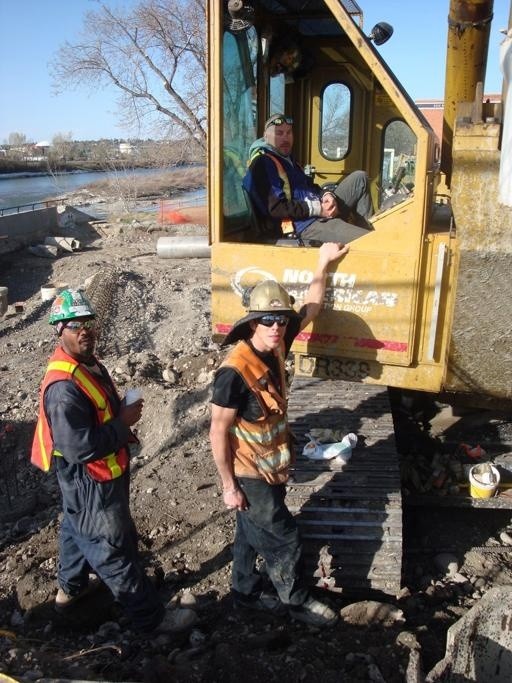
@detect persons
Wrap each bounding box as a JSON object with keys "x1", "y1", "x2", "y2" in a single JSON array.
[
  {"x1": 39, "y1": 287, "x2": 201, "y2": 634},
  {"x1": 207, "y1": 239, "x2": 349, "y2": 631},
  {"x1": 242, "y1": 110, "x2": 375, "y2": 248}
]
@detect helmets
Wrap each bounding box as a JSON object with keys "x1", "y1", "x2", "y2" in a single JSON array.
[
  {"x1": 48, "y1": 286, "x2": 95, "y2": 325},
  {"x1": 248, "y1": 276, "x2": 297, "y2": 314}
]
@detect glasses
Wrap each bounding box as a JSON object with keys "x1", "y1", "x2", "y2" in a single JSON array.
[
  {"x1": 271, "y1": 116, "x2": 295, "y2": 127},
  {"x1": 64, "y1": 320, "x2": 93, "y2": 331},
  {"x1": 252, "y1": 315, "x2": 290, "y2": 328}
]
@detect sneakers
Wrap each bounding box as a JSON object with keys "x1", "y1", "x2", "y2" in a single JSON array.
[
  {"x1": 233, "y1": 588, "x2": 288, "y2": 612},
  {"x1": 55, "y1": 590, "x2": 99, "y2": 613},
  {"x1": 154, "y1": 607, "x2": 197, "y2": 634},
  {"x1": 288, "y1": 599, "x2": 338, "y2": 627}
]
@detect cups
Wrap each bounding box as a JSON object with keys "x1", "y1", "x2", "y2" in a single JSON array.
[{"x1": 125, "y1": 389, "x2": 143, "y2": 406}]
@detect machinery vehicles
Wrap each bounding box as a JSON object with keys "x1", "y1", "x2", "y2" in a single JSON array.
[{"x1": 207, "y1": 0, "x2": 512, "y2": 606}]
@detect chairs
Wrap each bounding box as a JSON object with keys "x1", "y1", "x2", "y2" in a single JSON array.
[{"x1": 240, "y1": 185, "x2": 307, "y2": 246}]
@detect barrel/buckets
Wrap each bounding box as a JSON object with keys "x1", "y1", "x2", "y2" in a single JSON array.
[
  {"x1": 469, "y1": 465, "x2": 501, "y2": 498},
  {"x1": 0, "y1": 287, "x2": 8, "y2": 318},
  {"x1": 41, "y1": 282, "x2": 68, "y2": 302}
]
